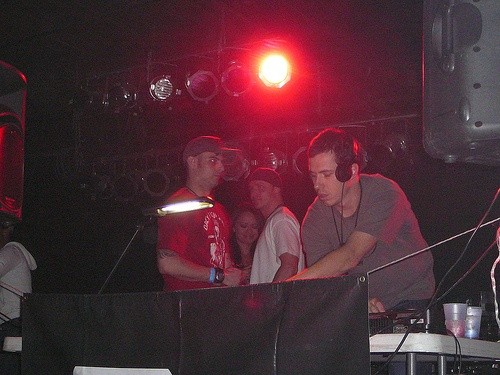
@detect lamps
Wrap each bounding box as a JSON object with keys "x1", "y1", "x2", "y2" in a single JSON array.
[
  {"x1": 95, "y1": 196, "x2": 215, "y2": 292},
  {"x1": 109, "y1": 45, "x2": 296, "y2": 112},
  {"x1": 143, "y1": 146, "x2": 308, "y2": 197}
]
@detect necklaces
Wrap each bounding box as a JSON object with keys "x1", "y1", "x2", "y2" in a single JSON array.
[
  {"x1": 263, "y1": 204, "x2": 287, "y2": 224},
  {"x1": 185, "y1": 186, "x2": 200, "y2": 198},
  {"x1": 331, "y1": 180, "x2": 362, "y2": 247}
]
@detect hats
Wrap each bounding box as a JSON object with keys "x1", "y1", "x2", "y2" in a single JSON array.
[
  {"x1": 183, "y1": 136, "x2": 243, "y2": 158},
  {"x1": 248, "y1": 169, "x2": 282, "y2": 188}
]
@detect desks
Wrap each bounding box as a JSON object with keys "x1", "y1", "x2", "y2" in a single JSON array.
[{"x1": 2, "y1": 333, "x2": 500, "y2": 375}]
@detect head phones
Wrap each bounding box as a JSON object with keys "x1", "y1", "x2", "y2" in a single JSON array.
[{"x1": 334, "y1": 133, "x2": 355, "y2": 182}]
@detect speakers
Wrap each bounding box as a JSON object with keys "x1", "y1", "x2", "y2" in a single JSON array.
[
  {"x1": 0, "y1": 60, "x2": 27, "y2": 228},
  {"x1": 422, "y1": 0, "x2": 500, "y2": 163}
]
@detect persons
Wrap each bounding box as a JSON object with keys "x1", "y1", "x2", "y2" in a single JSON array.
[
  {"x1": 247, "y1": 167, "x2": 306, "y2": 284},
  {"x1": 0, "y1": 211, "x2": 38, "y2": 375},
  {"x1": 156, "y1": 136, "x2": 242, "y2": 289},
  {"x1": 232, "y1": 204, "x2": 263, "y2": 285},
  {"x1": 285, "y1": 127, "x2": 438, "y2": 375}
]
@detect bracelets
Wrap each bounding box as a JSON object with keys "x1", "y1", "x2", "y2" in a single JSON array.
[
  {"x1": 209, "y1": 267, "x2": 215, "y2": 283},
  {"x1": 215, "y1": 267, "x2": 225, "y2": 284}
]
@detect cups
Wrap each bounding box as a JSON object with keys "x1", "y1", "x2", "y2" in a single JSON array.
[
  {"x1": 443, "y1": 303, "x2": 468, "y2": 338},
  {"x1": 464, "y1": 306, "x2": 483, "y2": 339}
]
